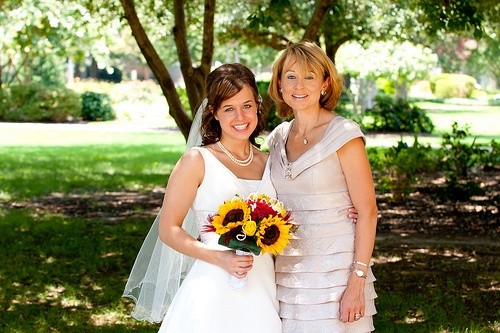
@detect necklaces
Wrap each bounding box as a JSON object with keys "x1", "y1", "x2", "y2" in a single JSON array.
[
  {"x1": 215, "y1": 136, "x2": 253, "y2": 167},
  {"x1": 293, "y1": 114, "x2": 318, "y2": 146}
]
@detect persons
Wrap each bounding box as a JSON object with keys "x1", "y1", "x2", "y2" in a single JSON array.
[
  {"x1": 158, "y1": 62, "x2": 357, "y2": 333},
  {"x1": 264, "y1": 41, "x2": 377, "y2": 333}
]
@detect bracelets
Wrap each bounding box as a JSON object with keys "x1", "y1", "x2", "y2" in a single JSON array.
[{"x1": 352, "y1": 260, "x2": 369, "y2": 269}]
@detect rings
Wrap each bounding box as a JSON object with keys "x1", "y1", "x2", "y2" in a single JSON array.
[{"x1": 355, "y1": 314, "x2": 361, "y2": 319}]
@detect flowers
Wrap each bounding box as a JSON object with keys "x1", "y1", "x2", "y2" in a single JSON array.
[{"x1": 200, "y1": 193, "x2": 301, "y2": 257}]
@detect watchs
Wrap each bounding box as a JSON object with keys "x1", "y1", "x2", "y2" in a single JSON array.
[{"x1": 350, "y1": 266, "x2": 367, "y2": 279}]
